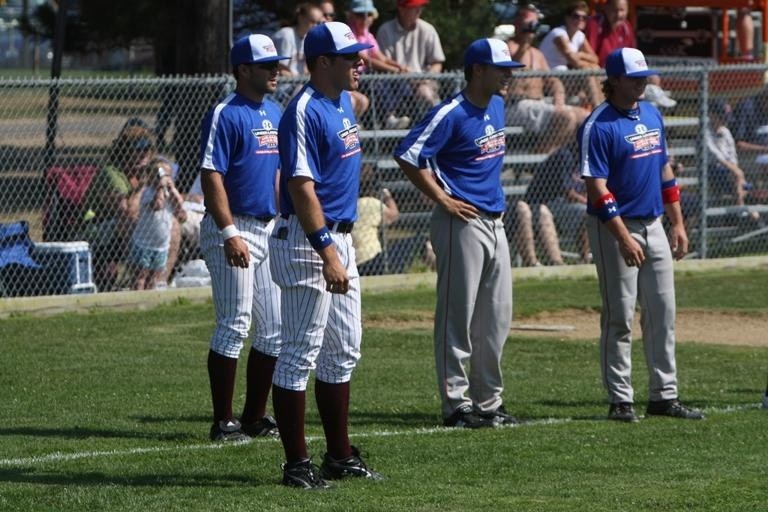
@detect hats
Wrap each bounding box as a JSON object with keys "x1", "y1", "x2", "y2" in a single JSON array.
[
  {"x1": 644, "y1": 84, "x2": 677, "y2": 108},
  {"x1": 465, "y1": 36, "x2": 527, "y2": 69},
  {"x1": 711, "y1": 103, "x2": 735, "y2": 122},
  {"x1": 604, "y1": 47, "x2": 662, "y2": 79},
  {"x1": 348, "y1": 1, "x2": 379, "y2": 19},
  {"x1": 229, "y1": 33, "x2": 293, "y2": 65},
  {"x1": 396, "y1": 0, "x2": 428, "y2": 7},
  {"x1": 303, "y1": 21, "x2": 375, "y2": 59}
]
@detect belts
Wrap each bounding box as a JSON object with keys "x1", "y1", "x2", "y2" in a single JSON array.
[
  {"x1": 254, "y1": 213, "x2": 271, "y2": 223},
  {"x1": 323, "y1": 218, "x2": 355, "y2": 234}
]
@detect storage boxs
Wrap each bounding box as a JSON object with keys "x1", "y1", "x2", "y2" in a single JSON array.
[{"x1": 33, "y1": 240, "x2": 98, "y2": 295}]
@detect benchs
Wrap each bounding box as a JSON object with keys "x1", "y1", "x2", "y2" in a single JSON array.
[{"x1": 359, "y1": 115, "x2": 740, "y2": 244}]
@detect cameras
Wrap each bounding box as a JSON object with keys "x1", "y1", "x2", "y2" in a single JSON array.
[{"x1": 526, "y1": 20, "x2": 549, "y2": 34}]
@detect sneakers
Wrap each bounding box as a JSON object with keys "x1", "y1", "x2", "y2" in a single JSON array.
[
  {"x1": 442, "y1": 403, "x2": 493, "y2": 430},
  {"x1": 280, "y1": 454, "x2": 330, "y2": 488},
  {"x1": 242, "y1": 415, "x2": 279, "y2": 438},
  {"x1": 479, "y1": 404, "x2": 519, "y2": 426},
  {"x1": 318, "y1": 445, "x2": 382, "y2": 481},
  {"x1": 209, "y1": 417, "x2": 250, "y2": 443},
  {"x1": 646, "y1": 397, "x2": 701, "y2": 420},
  {"x1": 607, "y1": 401, "x2": 640, "y2": 425}
]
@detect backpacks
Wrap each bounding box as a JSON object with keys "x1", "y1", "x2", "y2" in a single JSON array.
[{"x1": 0, "y1": 220, "x2": 69, "y2": 297}]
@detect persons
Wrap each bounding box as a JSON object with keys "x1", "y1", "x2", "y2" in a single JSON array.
[
  {"x1": 267, "y1": 19, "x2": 389, "y2": 493},
  {"x1": 392, "y1": 39, "x2": 522, "y2": 428},
  {"x1": 62, "y1": 1, "x2": 768, "y2": 292},
  {"x1": 578, "y1": 47, "x2": 702, "y2": 422},
  {"x1": 198, "y1": 32, "x2": 284, "y2": 446}
]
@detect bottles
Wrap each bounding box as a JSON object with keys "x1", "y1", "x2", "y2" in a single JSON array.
[{"x1": 153, "y1": 166, "x2": 170, "y2": 198}]
